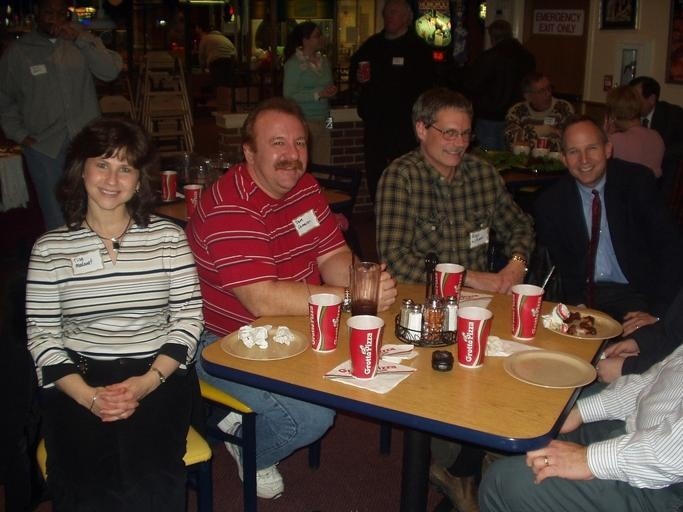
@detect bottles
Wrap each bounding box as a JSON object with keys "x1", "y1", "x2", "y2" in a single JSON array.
[
  {"x1": 399, "y1": 295, "x2": 458, "y2": 345},
  {"x1": 181, "y1": 154, "x2": 189, "y2": 183}
]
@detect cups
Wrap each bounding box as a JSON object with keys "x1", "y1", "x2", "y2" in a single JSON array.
[
  {"x1": 183, "y1": 183, "x2": 203, "y2": 219},
  {"x1": 359, "y1": 62, "x2": 371, "y2": 82},
  {"x1": 537, "y1": 137, "x2": 549, "y2": 148},
  {"x1": 510, "y1": 284, "x2": 545, "y2": 341},
  {"x1": 455, "y1": 305, "x2": 494, "y2": 369},
  {"x1": 309, "y1": 261, "x2": 385, "y2": 379},
  {"x1": 435, "y1": 262, "x2": 465, "y2": 308},
  {"x1": 160, "y1": 169, "x2": 178, "y2": 202}
]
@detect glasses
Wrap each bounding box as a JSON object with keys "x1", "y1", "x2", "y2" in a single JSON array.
[
  {"x1": 526, "y1": 85, "x2": 552, "y2": 94},
  {"x1": 426, "y1": 123, "x2": 472, "y2": 141}
]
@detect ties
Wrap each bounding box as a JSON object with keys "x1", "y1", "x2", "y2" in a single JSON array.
[
  {"x1": 642, "y1": 119, "x2": 649, "y2": 128},
  {"x1": 585, "y1": 189, "x2": 603, "y2": 309}
]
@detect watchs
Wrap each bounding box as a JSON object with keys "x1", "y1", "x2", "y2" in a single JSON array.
[
  {"x1": 150, "y1": 366, "x2": 167, "y2": 386},
  {"x1": 342, "y1": 285, "x2": 355, "y2": 315}
]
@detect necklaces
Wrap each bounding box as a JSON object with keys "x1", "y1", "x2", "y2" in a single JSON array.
[{"x1": 83, "y1": 212, "x2": 133, "y2": 253}]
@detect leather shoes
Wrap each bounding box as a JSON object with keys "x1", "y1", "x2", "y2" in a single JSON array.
[{"x1": 428, "y1": 463, "x2": 481, "y2": 512}]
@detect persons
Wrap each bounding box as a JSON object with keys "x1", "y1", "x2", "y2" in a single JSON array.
[
  {"x1": 576, "y1": 284, "x2": 683, "y2": 398},
  {"x1": 628, "y1": 76, "x2": 681, "y2": 193},
  {"x1": 184, "y1": 97, "x2": 398, "y2": 499},
  {"x1": 375, "y1": 87, "x2": 537, "y2": 295},
  {"x1": 507, "y1": 71, "x2": 572, "y2": 157},
  {"x1": 0, "y1": 0, "x2": 125, "y2": 235},
  {"x1": 529, "y1": 115, "x2": 682, "y2": 338},
  {"x1": 477, "y1": 345, "x2": 681, "y2": 511},
  {"x1": 470, "y1": 20, "x2": 534, "y2": 151},
  {"x1": 24, "y1": 115, "x2": 206, "y2": 510},
  {"x1": 349, "y1": 1, "x2": 435, "y2": 208},
  {"x1": 198, "y1": 29, "x2": 237, "y2": 79},
  {"x1": 602, "y1": 84, "x2": 666, "y2": 188},
  {"x1": 282, "y1": 20, "x2": 337, "y2": 179}
]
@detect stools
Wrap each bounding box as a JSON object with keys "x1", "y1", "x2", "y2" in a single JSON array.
[
  {"x1": 196, "y1": 375, "x2": 321, "y2": 511},
  {"x1": 139, "y1": 55, "x2": 195, "y2": 159},
  {"x1": 94, "y1": 77, "x2": 138, "y2": 123},
  {"x1": 37, "y1": 376, "x2": 215, "y2": 512}
]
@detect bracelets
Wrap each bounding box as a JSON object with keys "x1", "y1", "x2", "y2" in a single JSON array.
[
  {"x1": 86, "y1": 390, "x2": 100, "y2": 413},
  {"x1": 512, "y1": 255, "x2": 529, "y2": 264}
]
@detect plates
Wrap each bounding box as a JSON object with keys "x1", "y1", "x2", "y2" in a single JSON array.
[
  {"x1": 502, "y1": 348, "x2": 597, "y2": 390},
  {"x1": 221, "y1": 324, "x2": 310, "y2": 363},
  {"x1": 545, "y1": 303, "x2": 624, "y2": 342}
]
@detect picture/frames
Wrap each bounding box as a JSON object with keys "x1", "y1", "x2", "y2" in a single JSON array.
[{"x1": 598, "y1": 0, "x2": 640, "y2": 32}]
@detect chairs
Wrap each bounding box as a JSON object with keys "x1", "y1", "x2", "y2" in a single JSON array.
[{"x1": 308, "y1": 163, "x2": 362, "y2": 221}]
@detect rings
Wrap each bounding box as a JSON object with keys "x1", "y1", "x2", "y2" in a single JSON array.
[
  {"x1": 636, "y1": 325, "x2": 639, "y2": 331},
  {"x1": 596, "y1": 367, "x2": 600, "y2": 370},
  {"x1": 544, "y1": 455, "x2": 550, "y2": 467},
  {"x1": 634, "y1": 324, "x2": 639, "y2": 329}
]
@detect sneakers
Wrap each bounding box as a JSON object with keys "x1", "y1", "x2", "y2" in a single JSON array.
[{"x1": 224, "y1": 421, "x2": 285, "y2": 499}]
